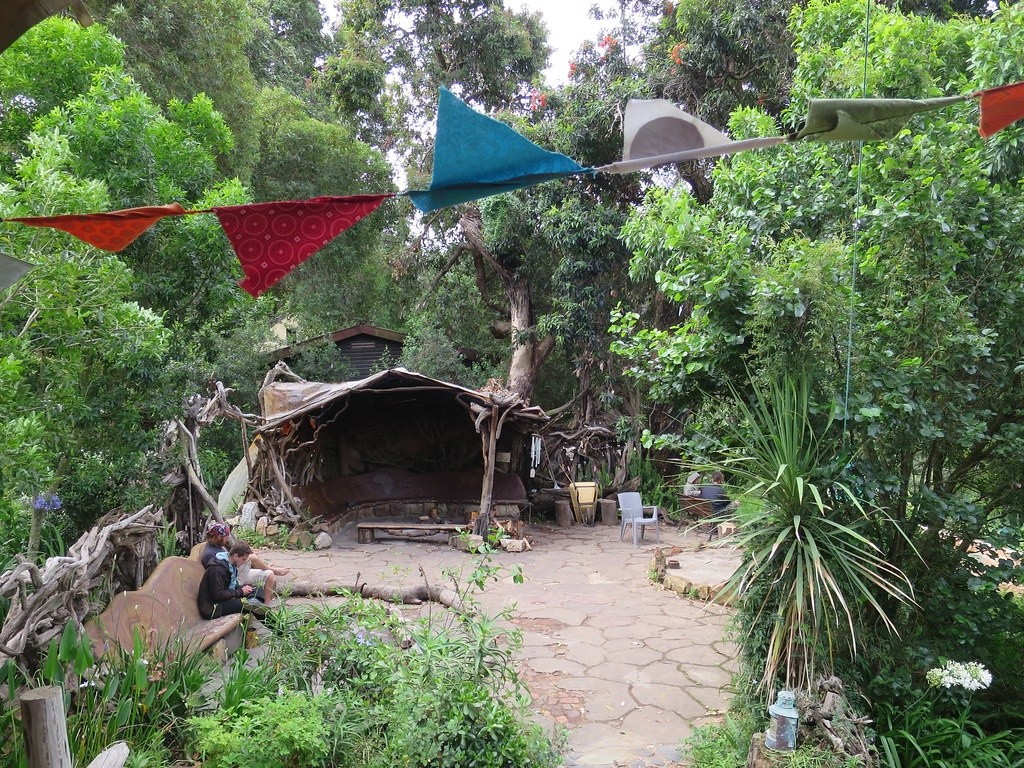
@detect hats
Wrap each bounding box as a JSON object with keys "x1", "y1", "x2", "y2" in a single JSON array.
[{"x1": 206, "y1": 522, "x2": 230, "y2": 537}]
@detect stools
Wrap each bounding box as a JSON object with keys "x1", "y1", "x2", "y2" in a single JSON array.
[
  {"x1": 598, "y1": 498, "x2": 619, "y2": 526},
  {"x1": 554, "y1": 499, "x2": 575, "y2": 528}
]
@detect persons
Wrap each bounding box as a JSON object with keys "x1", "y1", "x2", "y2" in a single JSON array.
[
  {"x1": 201, "y1": 525, "x2": 290, "y2": 605},
  {"x1": 197, "y1": 542, "x2": 284, "y2": 636},
  {"x1": 683, "y1": 471, "x2": 740, "y2": 521}
]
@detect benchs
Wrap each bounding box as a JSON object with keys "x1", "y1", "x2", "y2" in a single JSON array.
[
  {"x1": 676, "y1": 493, "x2": 716, "y2": 542},
  {"x1": 356, "y1": 522, "x2": 467, "y2": 547},
  {"x1": 82, "y1": 540, "x2": 249, "y2": 668},
  {"x1": 295, "y1": 471, "x2": 530, "y2": 539}
]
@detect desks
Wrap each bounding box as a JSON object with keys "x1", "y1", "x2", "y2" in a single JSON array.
[{"x1": 568, "y1": 482, "x2": 600, "y2": 526}]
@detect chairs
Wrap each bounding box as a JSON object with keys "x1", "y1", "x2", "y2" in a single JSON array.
[{"x1": 616, "y1": 492, "x2": 660, "y2": 547}]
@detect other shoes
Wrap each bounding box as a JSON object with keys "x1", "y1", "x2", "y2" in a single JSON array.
[
  {"x1": 287, "y1": 620, "x2": 303, "y2": 635},
  {"x1": 265, "y1": 600, "x2": 281, "y2": 608}
]
